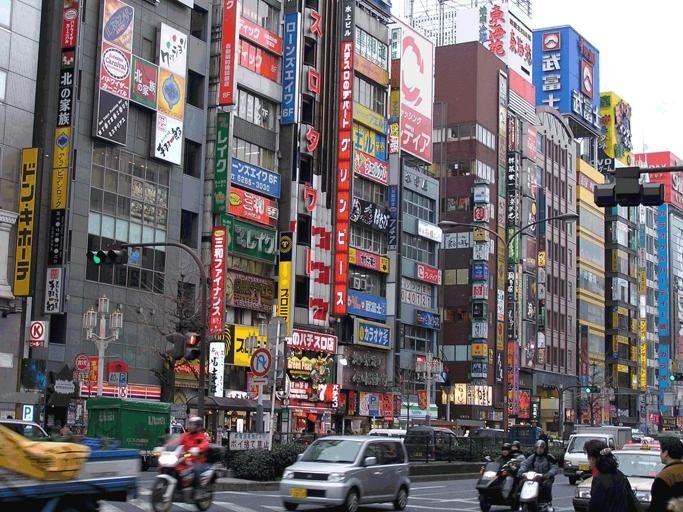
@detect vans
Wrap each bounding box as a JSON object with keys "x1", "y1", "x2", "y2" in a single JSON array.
[
  {"x1": 276, "y1": 435, "x2": 410, "y2": 510},
  {"x1": 403, "y1": 426, "x2": 459, "y2": 462},
  {"x1": 366, "y1": 427, "x2": 406, "y2": 438}
]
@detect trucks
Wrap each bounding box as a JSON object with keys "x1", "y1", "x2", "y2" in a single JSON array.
[
  {"x1": 0, "y1": 440, "x2": 141, "y2": 511},
  {"x1": 85, "y1": 396, "x2": 187, "y2": 471},
  {"x1": 563, "y1": 425, "x2": 631, "y2": 484},
  {"x1": 459, "y1": 425, "x2": 562, "y2": 446}
]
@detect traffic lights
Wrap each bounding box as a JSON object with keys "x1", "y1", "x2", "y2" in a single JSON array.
[
  {"x1": 164, "y1": 333, "x2": 184, "y2": 359},
  {"x1": 86, "y1": 249, "x2": 126, "y2": 264},
  {"x1": 540, "y1": 383, "x2": 556, "y2": 390},
  {"x1": 669, "y1": 373, "x2": 683, "y2": 381},
  {"x1": 185, "y1": 332, "x2": 200, "y2": 360},
  {"x1": 583, "y1": 386, "x2": 601, "y2": 394}
]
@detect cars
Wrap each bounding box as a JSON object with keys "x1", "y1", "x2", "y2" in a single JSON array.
[
  {"x1": 572, "y1": 441, "x2": 666, "y2": 512},
  {"x1": 622, "y1": 425, "x2": 683, "y2": 452},
  {"x1": 0, "y1": 417, "x2": 49, "y2": 439}
]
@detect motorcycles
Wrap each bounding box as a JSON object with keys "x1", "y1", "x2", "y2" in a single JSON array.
[
  {"x1": 476, "y1": 454, "x2": 520, "y2": 511},
  {"x1": 518, "y1": 469, "x2": 556, "y2": 512},
  {"x1": 149, "y1": 440, "x2": 226, "y2": 511}
]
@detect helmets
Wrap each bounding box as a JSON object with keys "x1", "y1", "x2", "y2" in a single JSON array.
[
  {"x1": 189, "y1": 417, "x2": 202, "y2": 434},
  {"x1": 502, "y1": 440, "x2": 546, "y2": 449}
]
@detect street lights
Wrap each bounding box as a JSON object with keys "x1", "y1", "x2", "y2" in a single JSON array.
[
  {"x1": 413, "y1": 351, "x2": 444, "y2": 421},
  {"x1": 82, "y1": 293, "x2": 124, "y2": 398},
  {"x1": 437, "y1": 213, "x2": 580, "y2": 450},
  {"x1": 441, "y1": 371, "x2": 453, "y2": 421}
]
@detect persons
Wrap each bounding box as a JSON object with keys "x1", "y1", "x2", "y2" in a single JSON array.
[
  {"x1": 480, "y1": 428, "x2": 558, "y2": 512},
  {"x1": 648, "y1": 437, "x2": 683, "y2": 512},
  {"x1": 583, "y1": 440, "x2": 641, "y2": 512},
  {"x1": 150, "y1": 415, "x2": 209, "y2": 501}
]
